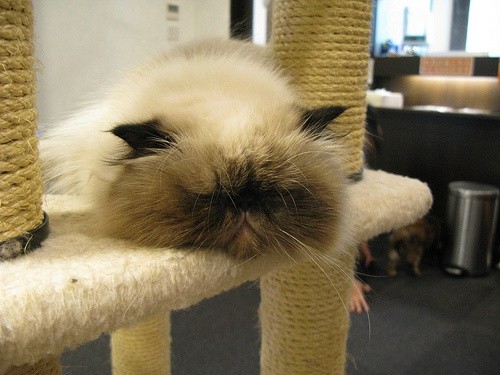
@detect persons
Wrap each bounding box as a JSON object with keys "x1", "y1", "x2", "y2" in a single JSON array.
[{"x1": 351, "y1": 106, "x2": 388, "y2": 315}]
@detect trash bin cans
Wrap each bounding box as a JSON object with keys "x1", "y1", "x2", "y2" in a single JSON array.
[{"x1": 439, "y1": 179, "x2": 500, "y2": 281}]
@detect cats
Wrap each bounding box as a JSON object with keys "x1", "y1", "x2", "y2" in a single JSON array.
[{"x1": 36, "y1": 34, "x2": 389, "y2": 345}]
